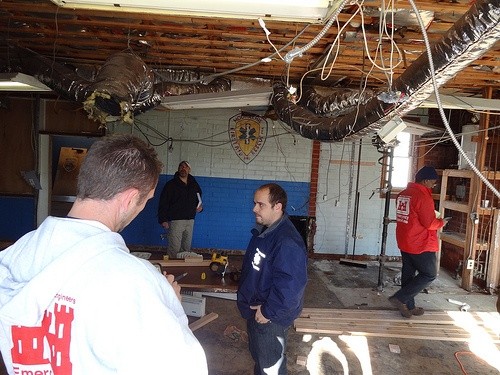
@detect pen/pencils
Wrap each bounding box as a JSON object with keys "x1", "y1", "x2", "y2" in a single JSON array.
[{"x1": 173, "y1": 272, "x2": 189, "y2": 282}]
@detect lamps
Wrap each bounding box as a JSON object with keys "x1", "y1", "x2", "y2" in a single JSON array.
[{"x1": 0, "y1": 72, "x2": 53, "y2": 91}]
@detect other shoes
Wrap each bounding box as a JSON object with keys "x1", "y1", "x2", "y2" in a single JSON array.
[
  {"x1": 388, "y1": 294, "x2": 412, "y2": 318},
  {"x1": 409, "y1": 307, "x2": 424, "y2": 316}
]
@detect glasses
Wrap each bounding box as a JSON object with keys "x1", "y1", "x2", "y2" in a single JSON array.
[{"x1": 427, "y1": 180, "x2": 437, "y2": 188}]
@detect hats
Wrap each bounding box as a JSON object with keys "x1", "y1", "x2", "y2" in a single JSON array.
[{"x1": 415, "y1": 166, "x2": 439, "y2": 180}]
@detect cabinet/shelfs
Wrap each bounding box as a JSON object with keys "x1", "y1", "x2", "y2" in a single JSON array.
[{"x1": 434, "y1": 169, "x2": 500, "y2": 292}]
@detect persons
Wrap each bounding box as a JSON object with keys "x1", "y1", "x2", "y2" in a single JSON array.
[
  {"x1": 388, "y1": 166, "x2": 453, "y2": 318},
  {"x1": 0, "y1": 135, "x2": 208, "y2": 375},
  {"x1": 236, "y1": 183, "x2": 307, "y2": 375}
]
